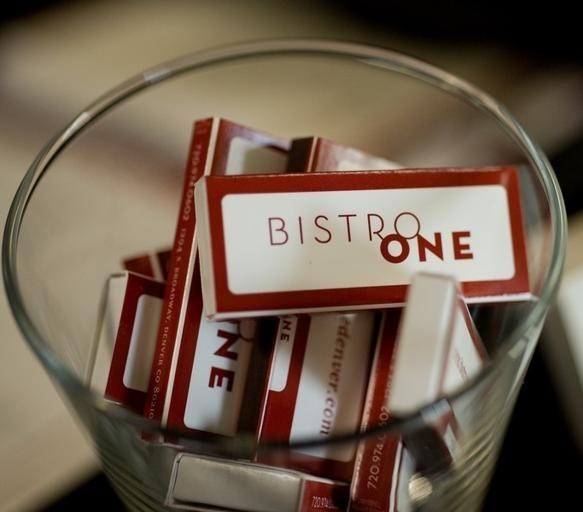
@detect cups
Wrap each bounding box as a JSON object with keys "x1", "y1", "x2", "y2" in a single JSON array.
[{"x1": 0, "y1": 38, "x2": 570, "y2": 511}]
